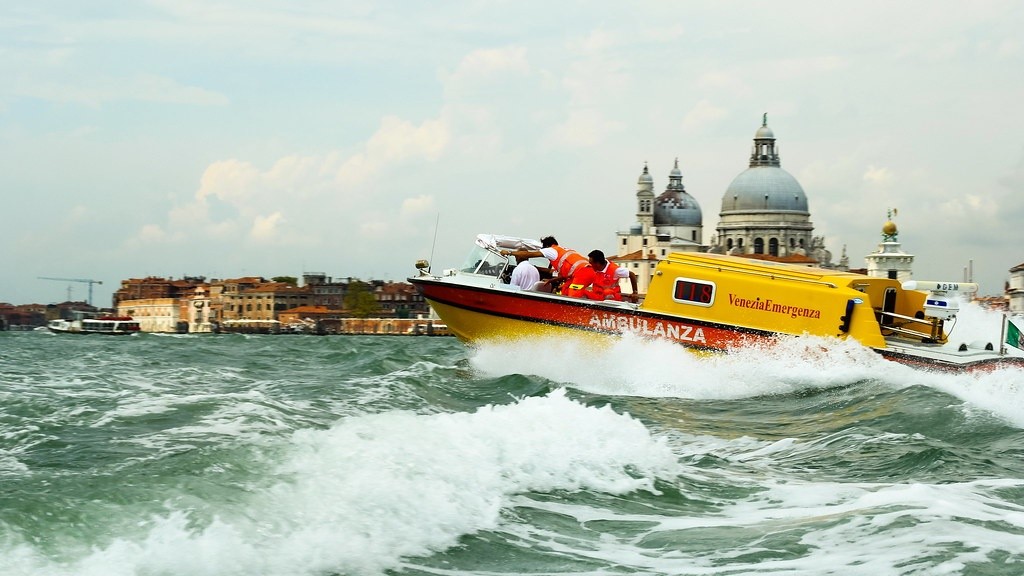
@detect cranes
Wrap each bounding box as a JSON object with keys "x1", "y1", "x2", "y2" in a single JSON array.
[{"x1": 37, "y1": 276, "x2": 104, "y2": 306}]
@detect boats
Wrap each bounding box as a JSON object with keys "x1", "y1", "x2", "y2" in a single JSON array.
[
  {"x1": 406, "y1": 231, "x2": 1024, "y2": 369},
  {"x1": 47, "y1": 317, "x2": 142, "y2": 334}
]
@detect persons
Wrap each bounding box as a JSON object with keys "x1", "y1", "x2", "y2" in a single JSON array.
[
  {"x1": 500, "y1": 236, "x2": 596, "y2": 298},
  {"x1": 510, "y1": 248, "x2": 540, "y2": 291},
  {"x1": 544, "y1": 250, "x2": 640, "y2": 304}
]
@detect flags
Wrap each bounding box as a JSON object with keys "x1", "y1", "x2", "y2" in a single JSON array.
[{"x1": 1005, "y1": 319, "x2": 1024, "y2": 350}]
